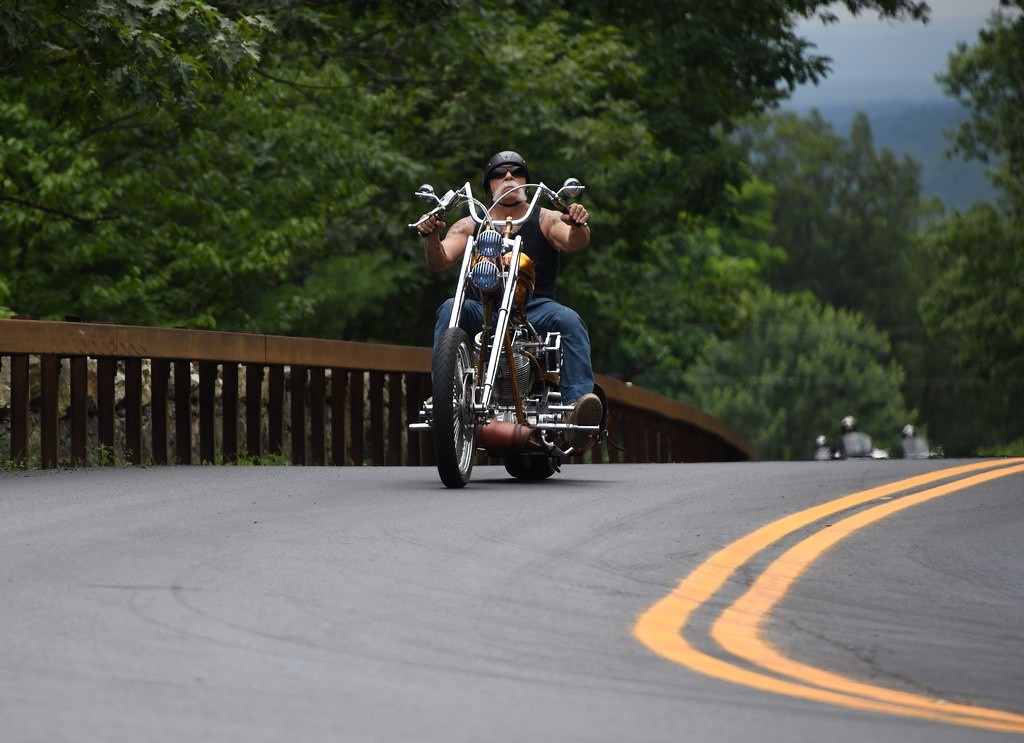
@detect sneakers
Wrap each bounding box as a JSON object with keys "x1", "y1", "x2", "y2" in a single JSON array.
[{"x1": 562, "y1": 393, "x2": 602, "y2": 457}]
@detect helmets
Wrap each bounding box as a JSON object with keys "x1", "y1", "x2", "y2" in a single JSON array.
[
  {"x1": 902, "y1": 424, "x2": 917, "y2": 438},
  {"x1": 840, "y1": 416, "x2": 857, "y2": 432},
  {"x1": 816, "y1": 435, "x2": 828, "y2": 447},
  {"x1": 483, "y1": 151, "x2": 529, "y2": 195}
]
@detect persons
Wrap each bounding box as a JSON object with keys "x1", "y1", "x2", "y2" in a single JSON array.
[
  {"x1": 903, "y1": 425, "x2": 916, "y2": 439},
  {"x1": 815, "y1": 436, "x2": 827, "y2": 447},
  {"x1": 417, "y1": 149, "x2": 603, "y2": 450},
  {"x1": 838, "y1": 417, "x2": 859, "y2": 436}
]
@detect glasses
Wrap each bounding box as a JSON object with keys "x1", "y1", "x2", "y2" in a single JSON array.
[{"x1": 488, "y1": 166, "x2": 527, "y2": 179}]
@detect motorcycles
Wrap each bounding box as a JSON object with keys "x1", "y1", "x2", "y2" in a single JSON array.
[
  {"x1": 814, "y1": 434, "x2": 889, "y2": 460},
  {"x1": 902, "y1": 436, "x2": 944, "y2": 460},
  {"x1": 406, "y1": 175, "x2": 602, "y2": 488}
]
